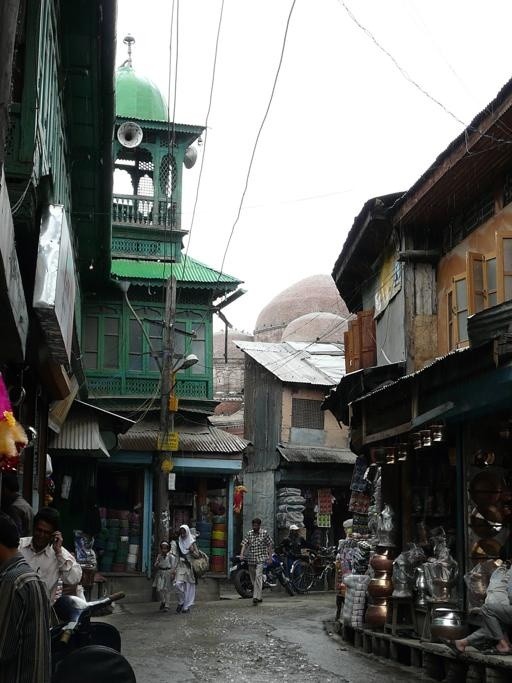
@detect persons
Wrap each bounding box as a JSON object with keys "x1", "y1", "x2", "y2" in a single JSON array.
[
  {"x1": 169, "y1": 525, "x2": 199, "y2": 612},
  {"x1": 280, "y1": 524, "x2": 320, "y2": 594},
  {"x1": 239, "y1": 517, "x2": 272, "y2": 603},
  {"x1": 0, "y1": 470, "x2": 87, "y2": 683},
  {"x1": 153, "y1": 540, "x2": 172, "y2": 611},
  {"x1": 442, "y1": 605, "x2": 512, "y2": 655}
]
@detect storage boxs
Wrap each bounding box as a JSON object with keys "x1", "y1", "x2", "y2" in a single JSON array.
[{"x1": 193, "y1": 521, "x2": 226, "y2": 571}]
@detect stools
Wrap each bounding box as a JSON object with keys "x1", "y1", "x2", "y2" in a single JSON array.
[{"x1": 382, "y1": 592, "x2": 418, "y2": 638}]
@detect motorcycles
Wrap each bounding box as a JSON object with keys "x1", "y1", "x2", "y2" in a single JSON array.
[
  {"x1": 228, "y1": 536, "x2": 298, "y2": 598},
  {"x1": 49, "y1": 587, "x2": 136, "y2": 683}
]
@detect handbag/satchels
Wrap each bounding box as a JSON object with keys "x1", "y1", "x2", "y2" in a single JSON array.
[{"x1": 192, "y1": 550, "x2": 210, "y2": 577}]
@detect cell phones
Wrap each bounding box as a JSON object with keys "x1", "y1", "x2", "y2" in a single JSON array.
[{"x1": 48, "y1": 534, "x2": 58, "y2": 547}]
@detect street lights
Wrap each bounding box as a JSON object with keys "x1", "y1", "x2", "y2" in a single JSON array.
[{"x1": 154, "y1": 353, "x2": 201, "y2": 572}]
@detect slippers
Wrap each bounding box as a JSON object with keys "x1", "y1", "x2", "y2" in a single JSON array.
[
  {"x1": 438, "y1": 635, "x2": 460, "y2": 656},
  {"x1": 481, "y1": 646, "x2": 509, "y2": 655}
]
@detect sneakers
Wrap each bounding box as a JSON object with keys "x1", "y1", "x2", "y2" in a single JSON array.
[
  {"x1": 176, "y1": 605, "x2": 183, "y2": 613},
  {"x1": 160, "y1": 602, "x2": 165, "y2": 610},
  {"x1": 253, "y1": 598, "x2": 258, "y2": 603}
]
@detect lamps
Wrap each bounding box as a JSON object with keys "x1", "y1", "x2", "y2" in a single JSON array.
[{"x1": 197, "y1": 134, "x2": 203, "y2": 145}]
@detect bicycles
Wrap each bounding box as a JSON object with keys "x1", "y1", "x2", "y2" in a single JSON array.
[{"x1": 289, "y1": 546, "x2": 338, "y2": 591}]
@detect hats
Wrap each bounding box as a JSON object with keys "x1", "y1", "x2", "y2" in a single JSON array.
[{"x1": 290, "y1": 525, "x2": 300, "y2": 530}]
